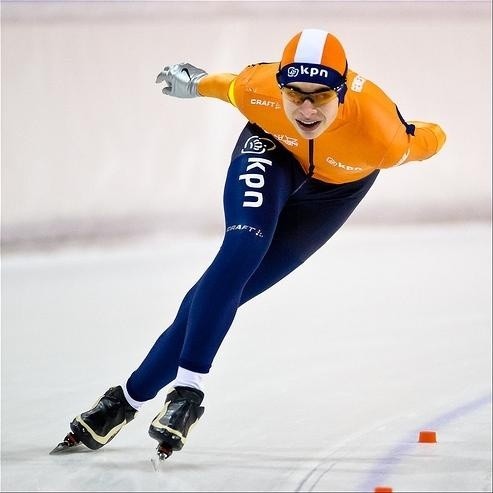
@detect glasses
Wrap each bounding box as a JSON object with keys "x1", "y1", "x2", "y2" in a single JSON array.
[{"x1": 276, "y1": 82, "x2": 345, "y2": 107}]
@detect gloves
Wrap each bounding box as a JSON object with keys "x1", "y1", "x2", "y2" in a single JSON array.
[{"x1": 154, "y1": 62, "x2": 208, "y2": 99}]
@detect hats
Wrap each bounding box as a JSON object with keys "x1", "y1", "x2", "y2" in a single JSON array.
[{"x1": 274, "y1": 29, "x2": 349, "y2": 105}]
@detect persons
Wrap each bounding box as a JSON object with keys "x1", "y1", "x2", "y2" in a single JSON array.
[{"x1": 68, "y1": 25, "x2": 448, "y2": 454}]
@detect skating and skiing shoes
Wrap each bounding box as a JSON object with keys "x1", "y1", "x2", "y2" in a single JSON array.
[
  {"x1": 147, "y1": 385, "x2": 206, "y2": 472},
  {"x1": 45, "y1": 384, "x2": 138, "y2": 455}
]
